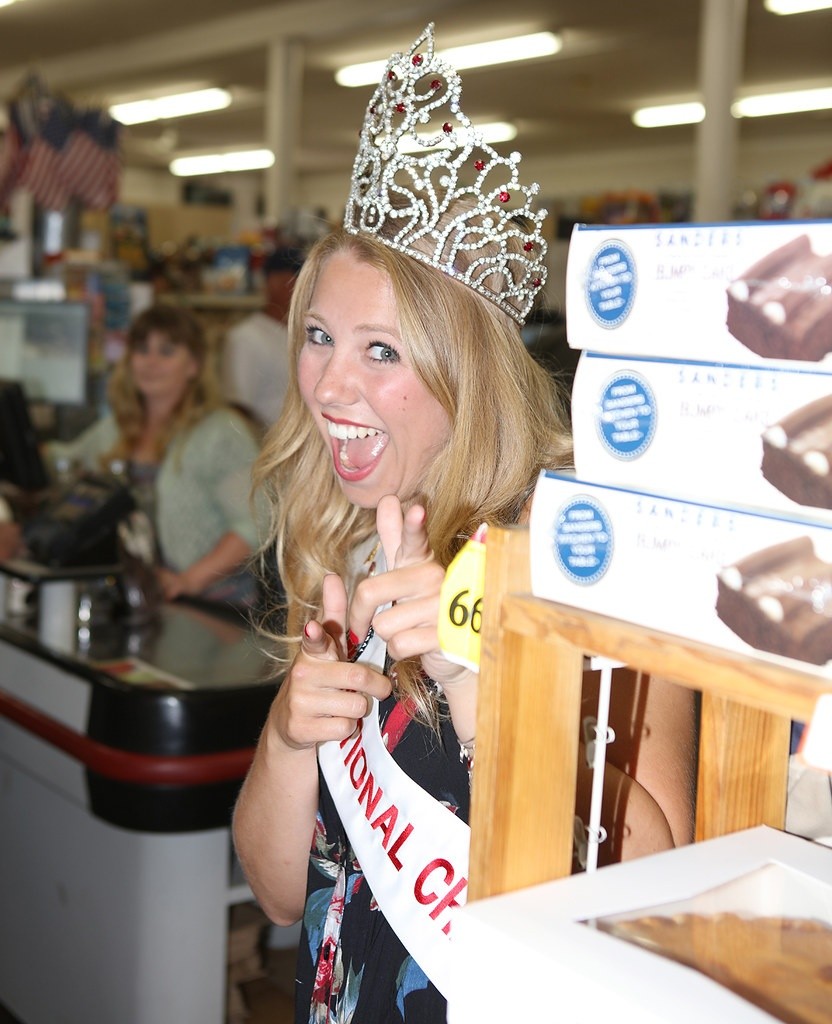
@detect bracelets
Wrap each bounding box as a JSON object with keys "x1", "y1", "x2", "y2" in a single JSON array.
[{"x1": 456, "y1": 737, "x2": 475, "y2": 794}]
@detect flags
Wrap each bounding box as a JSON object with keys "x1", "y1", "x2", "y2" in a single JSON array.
[{"x1": 20, "y1": 93, "x2": 123, "y2": 217}]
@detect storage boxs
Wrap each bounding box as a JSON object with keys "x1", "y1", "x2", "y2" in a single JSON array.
[
  {"x1": 452, "y1": 824, "x2": 832, "y2": 1024},
  {"x1": 526, "y1": 222, "x2": 832, "y2": 676}
]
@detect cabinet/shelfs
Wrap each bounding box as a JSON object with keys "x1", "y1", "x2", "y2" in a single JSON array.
[{"x1": 465, "y1": 528, "x2": 832, "y2": 899}]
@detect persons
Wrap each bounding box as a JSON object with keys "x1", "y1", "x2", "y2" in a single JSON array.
[
  {"x1": 0, "y1": 123, "x2": 30, "y2": 205},
  {"x1": 38, "y1": 304, "x2": 275, "y2": 634},
  {"x1": 229, "y1": 192, "x2": 695, "y2": 1024},
  {"x1": 223, "y1": 244, "x2": 304, "y2": 425}
]
